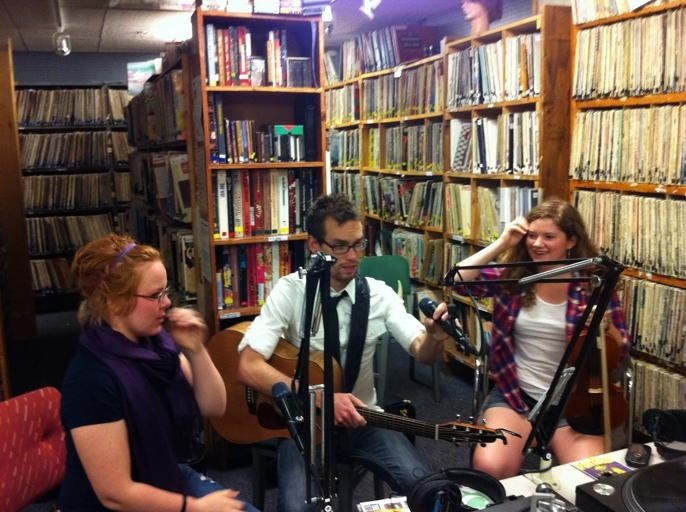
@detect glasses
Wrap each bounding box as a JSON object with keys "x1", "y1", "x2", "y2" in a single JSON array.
[
  {"x1": 132, "y1": 285, "x2": 171, "y2": 302},
  {"x1": 322, "y1": 239, "x2": 368, "y2": 254}
]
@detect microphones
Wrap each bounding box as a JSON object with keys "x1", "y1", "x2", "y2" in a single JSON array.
[
  {"x1": 419, "y1": 298, "x2": 475, "y2": 355},
  {"x1": 271, "y1": 382, "x2": 308, "y2": 455}
]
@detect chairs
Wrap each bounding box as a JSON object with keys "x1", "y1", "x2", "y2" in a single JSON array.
[{"x1": 1, "y1": 385, "x2": 70, "y2": 512}]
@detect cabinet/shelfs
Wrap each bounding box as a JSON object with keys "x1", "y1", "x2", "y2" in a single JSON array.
[{"x1": 0, "y1": 5, "x2": 686, "y2": 452}]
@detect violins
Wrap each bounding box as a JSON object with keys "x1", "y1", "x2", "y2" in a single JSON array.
[{"x1": 565, "y1": 266, "x2": 628, "y2": 434}]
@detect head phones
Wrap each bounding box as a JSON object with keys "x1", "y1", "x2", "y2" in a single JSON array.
[{"x1": 405, "y1": 467, "x2": 506, "y2": 511}]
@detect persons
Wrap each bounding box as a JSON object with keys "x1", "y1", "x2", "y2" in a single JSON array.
[
  {"x1": 452, "y1": 199, "x2": 631, "y2": 481},
  {"x1": 60, "y1": 235, "x2": 256, "y2": 512},
  {"x1": 237, "y1": 195, "x2": 459, "y2": 512}
]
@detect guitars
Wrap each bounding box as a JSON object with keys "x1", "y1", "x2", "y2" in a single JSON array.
[{"x1": 205, "y1": 322, "x2": 508, "y2": 447}]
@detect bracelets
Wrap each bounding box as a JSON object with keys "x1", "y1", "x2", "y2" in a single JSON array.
[{"x1": 178, "y1": 491, "x2": 188, "y2": 509}]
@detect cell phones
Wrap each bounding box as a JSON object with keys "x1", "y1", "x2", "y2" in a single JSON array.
[{"x1": 625, "y1": 441, "x2": 651, "y2": 468}]
